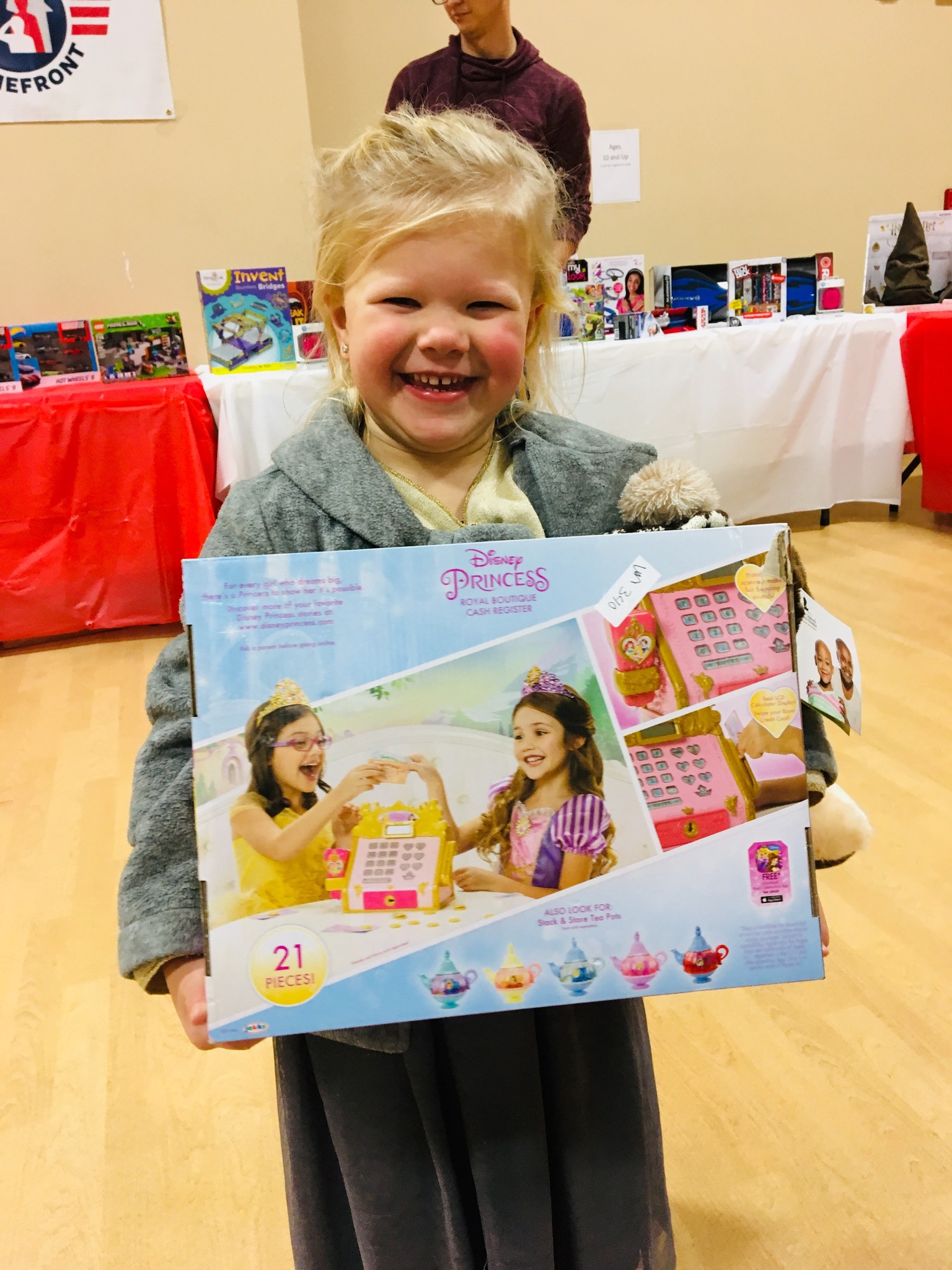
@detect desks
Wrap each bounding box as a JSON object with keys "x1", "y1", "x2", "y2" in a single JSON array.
[{"x1": 3, "y1": 301, "x2": 950, "y2": 655}]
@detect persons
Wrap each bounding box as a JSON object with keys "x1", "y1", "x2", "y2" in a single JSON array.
[
  {"x1": 616, "y1": 267, "x2": 644, "y2": 315},
  {"x1": 380, "y1": 0, "x2": 599, "y2": 275},
  {"x1": 737, "y1": 717, "x2": 807, "y2": 810},
  {"x1": 806, "y1": 638, "x2": 861, "y2": 735},
  {"x1": 806, "y1": 638, "x2": 845, "y2": 720},
  {"x1": 397, "y1": 677, "x2": 618, "y2": 900},
  {"x1": 206, "y1": 696, "x2": 383, "y2": 923},
  {"x1": 112, "y1": 103, "x2": 840, "y2": 1270}
]
